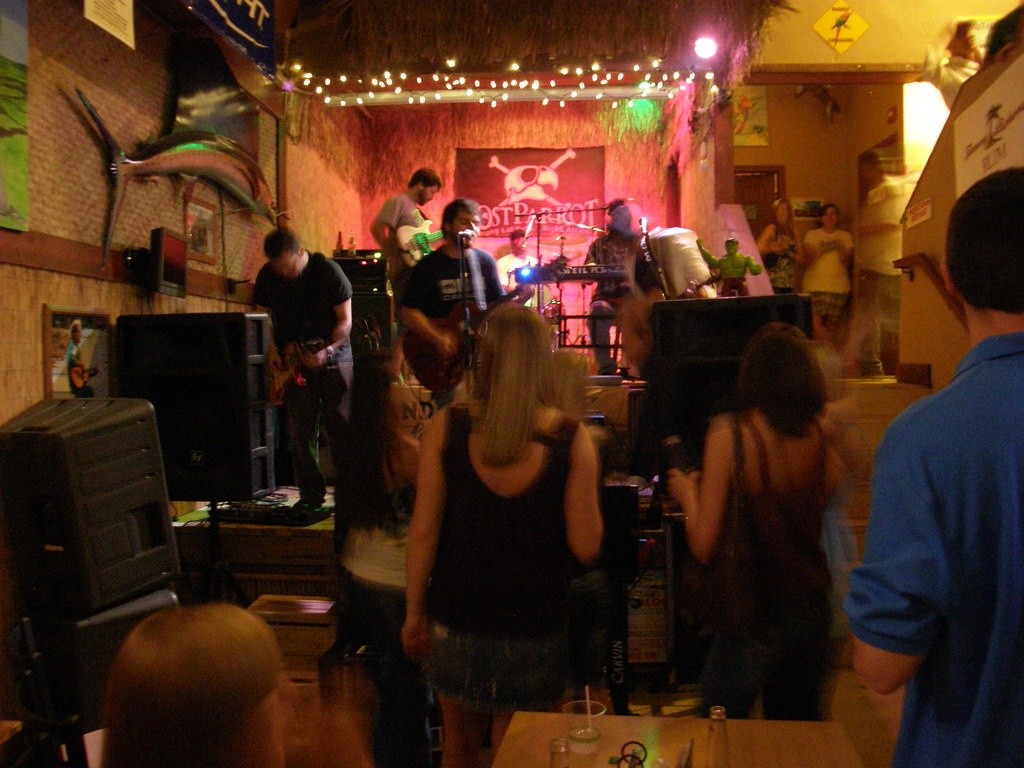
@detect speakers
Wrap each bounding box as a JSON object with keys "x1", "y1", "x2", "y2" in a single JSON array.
[
  {"x1": 114, "y1": 313, "x2": 276, "y2": 502},
  {"x1": 10, "y1": 589, "x2": 180, "y2": 739},
  {"x1": 0, "y1": 399, "x2": 181, "y2": 618},
  {"x1": 651, "y1": 294, "x2": 813, "y2": 497},
  {"x1": 350, "y1": 295, "x2": 391, "y2": 349}
]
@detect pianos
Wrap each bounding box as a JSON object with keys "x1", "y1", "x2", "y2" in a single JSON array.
[{"x1": 514, "y1": 264, "x2": 628, "y2": 373}]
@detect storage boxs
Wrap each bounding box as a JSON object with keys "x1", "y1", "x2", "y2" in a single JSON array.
[{"x1": 246, "y1": 594, "x2": 336, "y2": 682}]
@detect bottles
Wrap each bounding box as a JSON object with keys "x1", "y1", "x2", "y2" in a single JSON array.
[
  {"x1": 348, "y1": 237, "x2": 356, "y2": 257},
  {"x1": 549, "y1": 738, "x2": 570, "y2": 768},
  {"x1": 337, "y1": 231, "x2": 343, "y2": 257},
  {"x1": 706, "y1": 705, "x2": 730, "y2": 768}
]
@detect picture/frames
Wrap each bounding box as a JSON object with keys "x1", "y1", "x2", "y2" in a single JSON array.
[
  {"x1": 181, "y1": 193, "x2": 219, "y2": 265},
  {"x1": 41, "y1": 302, "x2": 117, "y2": 400}
]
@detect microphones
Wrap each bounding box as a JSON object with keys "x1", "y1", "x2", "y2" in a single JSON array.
[
  {"x1": 457, "y1": 229, "x2": 475, "y2": 239},
  {"x1": 639, "y1": 217, "x2": 648, "y2": 234}
]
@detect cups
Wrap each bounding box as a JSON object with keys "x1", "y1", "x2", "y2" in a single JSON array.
[{"x1": 563, "y1": 700, "x2": 607, "y2": 763}]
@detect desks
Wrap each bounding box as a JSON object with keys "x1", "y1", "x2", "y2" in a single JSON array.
[
  {"x1": 491, "y1": 711, "x2": 863, "y2": 768},
  {"x1": 173, "y1": 487, "x2": 335, "y2": 607}
]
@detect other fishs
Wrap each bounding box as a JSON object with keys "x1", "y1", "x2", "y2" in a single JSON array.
[{"x1": 72, "y1": 83, "x2": 280, "y2": 274}]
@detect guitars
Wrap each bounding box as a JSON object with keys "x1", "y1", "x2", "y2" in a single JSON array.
[
  {"x1": 395, "y1": 218, "x2": 444, "y2": 268},
  {"x1": 401, "y1": 253, "x2": 571, "y2": 393},
  {"x1": 70, "y1": 366, "x2": 98, "y2": 389},
  {"x1": 267, "y1": 333, "x2": 349, "y2": 407}
]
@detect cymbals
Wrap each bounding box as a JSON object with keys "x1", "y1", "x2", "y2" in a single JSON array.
[{"x1": 538, "y1": 233, "x2": 589, "y2": 247}]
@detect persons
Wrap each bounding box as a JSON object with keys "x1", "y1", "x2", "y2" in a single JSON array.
[
  {"x1": 758, "y1": 147, "x2": 907, "y2": 380},
  {"x1": 99, "y1": 603, "x2": 289, "y2": 768},
  {"x1": 369, "y1": 167, "x2": 721, "y2": 409},
  {"x1": 667, "y1": 323, "x2": 843, "y2": 721},
  {"x1": 844, "y1": 166, "x2": 1024, "y2": 768},
  {"x1": 252, "y1": 225, "x2": 354, "y2": 513},
  {"x1": 921, "y1": 16, "x2": 987, "y2": 113},
  {"x1": 696, "y1": 236, "x2": 764, "y2": 297},
  {"x1": 66, "y1": 320, "x2": 100, "y2": 397},
  {"x1": 403, "y1": 303, "x2": 603, "y2": 768}
]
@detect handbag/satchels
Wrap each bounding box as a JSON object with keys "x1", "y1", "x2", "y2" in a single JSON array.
[{"x1": 691, "y1": 402, "x2": 772, "y2": 638}]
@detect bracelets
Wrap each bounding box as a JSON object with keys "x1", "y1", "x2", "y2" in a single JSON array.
[
  {"x1": 684, "y1": 517, "x2": 696, "y2": 521},
  {"x1": 407, "y1": 607, "x2": 423, "y2": 615},
  {"x1": 327, "y1": 346, "x2": 334, "y2": 357}
]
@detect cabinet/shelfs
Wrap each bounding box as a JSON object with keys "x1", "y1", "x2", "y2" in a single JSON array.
[
  {"x1": 847, "y1": 126, "x2": 901, "y2": 360},
  {"x1": 628, "y1": 497, "x2": 684, "y2": 665}
]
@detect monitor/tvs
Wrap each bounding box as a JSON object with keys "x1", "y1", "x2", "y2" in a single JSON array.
[{"x1": 148, "y1": 226, "x2": 189, "y2": 298}]
